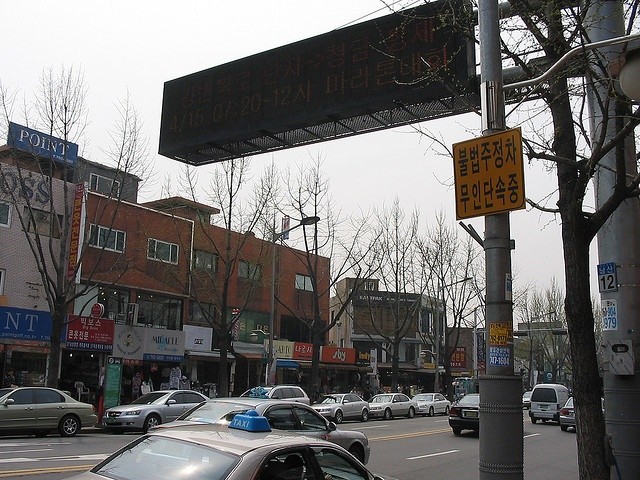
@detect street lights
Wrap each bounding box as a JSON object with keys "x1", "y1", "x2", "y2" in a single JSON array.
[
  {"x1": 248, "y1": 328, "x2": 270, "y2": 340},
  {"x1": 419, "y1": 348, "x2": 437, "y2": 359},
  {"x1": 434, "y1": 276, "x2": 473, "y2": 391},
  {"x1": 267, "y1": 215, "x2": 321, "y2": 384},
  {"x1": 475, "y1": 29, "x2": 640, "y2": 480},
  {"x1": 529, "y1": 311, "x2": 555, "y2": 384}
]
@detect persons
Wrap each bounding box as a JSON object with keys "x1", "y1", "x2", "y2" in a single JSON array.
[
  {"x1": 276, "y1": 436, "x2": 313, "y2": 471},
  {"x1": 402, "y1": 386, "x2": 409, "y2": 396},
  {"x1": 363, "y1": 387, "x2": 371, "y2": 401},
  {"x1": 349, "y1": 386, "x2": 358, "y2": 393}
]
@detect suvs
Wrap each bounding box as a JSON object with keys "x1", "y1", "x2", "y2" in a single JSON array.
[{"x1": 239, "y1": 384, "x2": 311, "y2": 418}]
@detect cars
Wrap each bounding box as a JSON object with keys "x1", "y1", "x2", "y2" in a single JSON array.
[
  {"x1": 0, "y1": 387, "x2": 98, "y2": 437},
  {"x1": 412, "y1": 392, "x2": 451, "y2": 417},
  {"x1": 368, "y1": 392, "x2": 419, "y2": 419},
  {"x1": 102, "y1": 389, "x2": 211, "y2": 434},
  {"x1": 448, "y1": 393, "x2": 480, "y2": 435},
  {"x1": 311, "y1": 393, "x2": 370, "y2": 423},
  {"x1": 523, "y1": 391, "x2": 533, "y2": 408},
  {"x1": 559, "y1": 396, "x2": 605, "y2": 431},
  {"x1": 62, "y1": 410, "x2": 401, "y2": 480},
  {"x1": 148, "y1": 397, "x2": 370, "y2": 466}
]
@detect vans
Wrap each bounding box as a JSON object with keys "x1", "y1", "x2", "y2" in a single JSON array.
[{"x1": 529, "y1": 383, "x2": 570, "y2": 424}]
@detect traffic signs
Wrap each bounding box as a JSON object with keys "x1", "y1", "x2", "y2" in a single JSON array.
[{"x1": 452, "y1": 128, "x2": 523, "y2": 219}]
